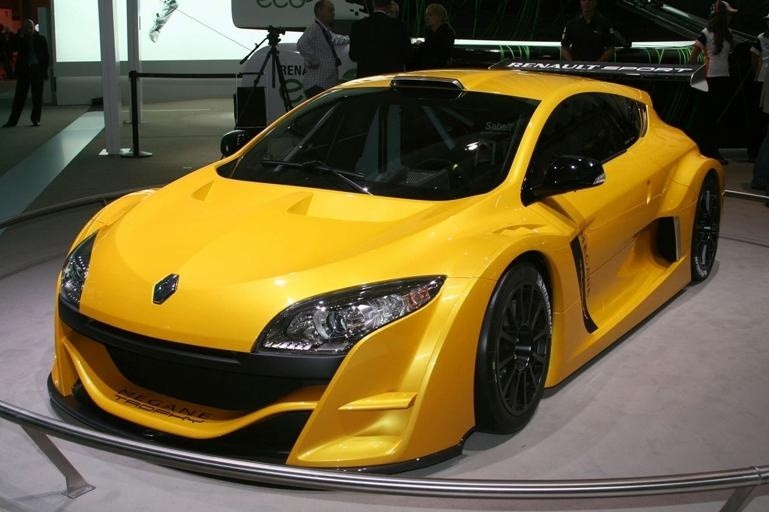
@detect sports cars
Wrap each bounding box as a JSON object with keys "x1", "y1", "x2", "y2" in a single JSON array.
[{"x1": 48, "y1": 67, "x2": 726, "y2": 477}]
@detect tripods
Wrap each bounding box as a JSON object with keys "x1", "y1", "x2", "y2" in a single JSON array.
[{"x1": 221, "y1": 48, "x2": 293, "y2": 160}]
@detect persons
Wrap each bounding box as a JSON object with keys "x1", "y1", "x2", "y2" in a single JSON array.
[
  {"x1": 417, "y1": 3, "x2": 457, "y2": 70},
  {"x1": 560, "y1": 0, "x2": 615, "y2": 63},
  {"x1": 2, "y1": 18, "x2": 50, "y2": 127},
  {"x1": 746, "y1": 12, "x2": 769, "y2": 163},
  {"x1": 0, "y1": 23, "x2": 16, "y2": 80},
  {"x1": 386, "y1": 1, "x2": 399, "y2": 17},
  {"x1": 296, "y1": 0, "x2": 351, "y2": 100},
  {"x1": 688, "y1": 0, "x2": 739, "y2": 165},
  {"x1": 750, "y1": 61, "x2": 769, "y2": 190},
  {"x1": 348, "y1": 0, "x2": 408, "y2": 79}
]
